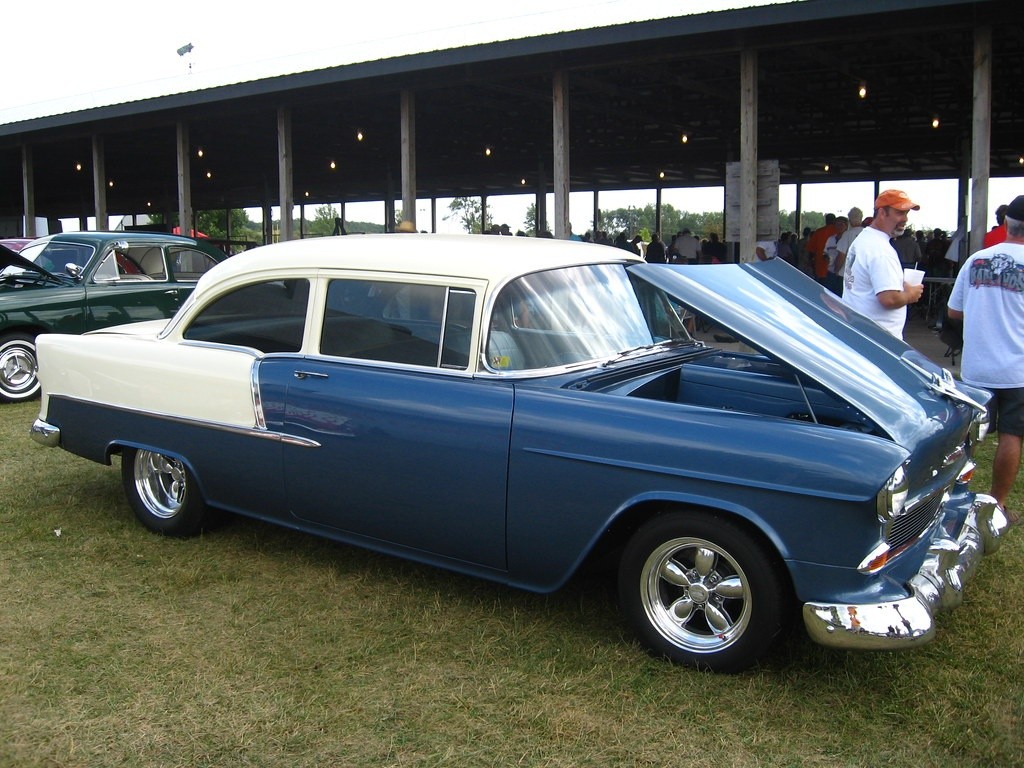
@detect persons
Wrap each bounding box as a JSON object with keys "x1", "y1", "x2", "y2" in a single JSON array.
[
  {"x1": 840, "y1": 189, "x2": 924, "y2": 340},
  {"x1": 946, "y1": 195, "x2": 1024, "y2": 525},
  {"x1": 483, "y1": 208, "x2": 1009, "y2": 319}
]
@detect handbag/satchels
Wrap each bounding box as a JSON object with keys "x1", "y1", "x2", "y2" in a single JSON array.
[
  {"x1": 943, "y1": 218, "x2": 965, "y2": 262},
  {"x1": 937, "y1": 318, "x2": 963, "y2": 366}
]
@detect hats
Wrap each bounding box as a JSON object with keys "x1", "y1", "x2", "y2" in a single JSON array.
[
  {"x1": 904, "y1": 229, "x2": 914, "y2": 235},
  {"x1": 1002, "y1": 195, "x2": 1024, "y2": 221},
  {"x1": 848, "y1": 207, "x2": 863, "y2": 226},
  {"x1": 874, "y1": 189, "x2": 920, "y2": 211},
  {"x1": 683, "y1": 228, "x2": 689, "y2": 232},
  {"x1": 636, "y1": 235, "x2": 643, "y2": 241}
]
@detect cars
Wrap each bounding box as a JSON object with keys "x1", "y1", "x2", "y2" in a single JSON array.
[
  {"x1": 0, "y1": 238, "x2": 143, "y2": 278},
  {"x1": 0, "y1": 230, "x2": 288, "y2": 404},
  {"x1": 29, "y1": 231, "x2": 1008, "y2": 669}
]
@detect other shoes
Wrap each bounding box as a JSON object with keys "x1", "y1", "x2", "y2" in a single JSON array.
[{"x1": 1003, "y1": 505, "x2": 1021, "y2": 525}]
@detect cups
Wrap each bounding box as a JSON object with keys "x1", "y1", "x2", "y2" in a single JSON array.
[{"x1": 903, "y1": 268, "x2": 925, "y2": 303}]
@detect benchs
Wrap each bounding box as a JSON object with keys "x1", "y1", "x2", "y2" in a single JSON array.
[{"x1": 201, "y1": 315, "x2": 393, "y2": 358}]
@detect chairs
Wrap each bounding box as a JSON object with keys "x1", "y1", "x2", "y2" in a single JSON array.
[{"x1": 449, "y1": 330, "x2": 527, "y2": 379}]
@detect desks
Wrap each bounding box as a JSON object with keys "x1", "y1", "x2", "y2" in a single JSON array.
[{"x1": 922, "y1": 277, "x2": 956, "y2": 321}]
